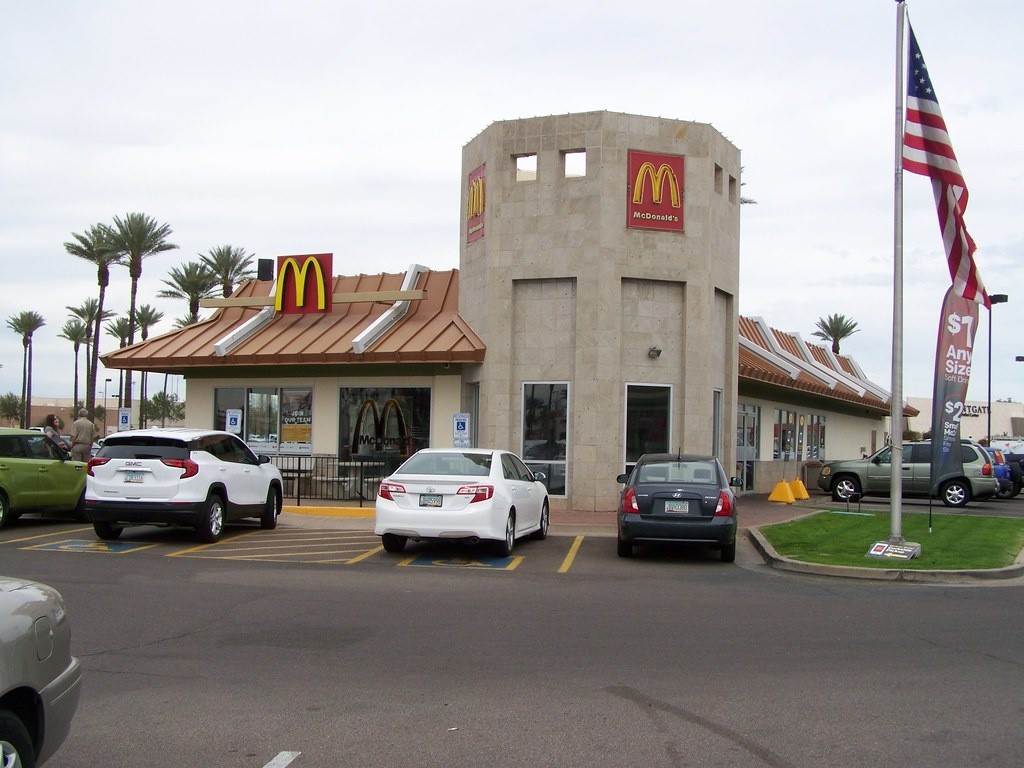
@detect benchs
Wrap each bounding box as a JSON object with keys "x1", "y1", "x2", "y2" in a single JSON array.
[{"x1": 312, "y1": 477, "x2": 385, "y2": 500}]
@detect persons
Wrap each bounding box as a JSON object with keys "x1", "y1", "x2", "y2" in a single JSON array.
[
  {"x1": 43, "y1": 412, "x2": 72, "y2": 463},
  {"x1": 70, "y1": 407, "x2": 95, "y2": 462}
]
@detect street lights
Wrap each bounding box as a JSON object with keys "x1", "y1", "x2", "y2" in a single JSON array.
[
  {"x1": 987, "y1": 294, "x2": 1008, "y2": 448},
  {"x1": 103, "y1": 379, "x2": 111, "y2": 437}
]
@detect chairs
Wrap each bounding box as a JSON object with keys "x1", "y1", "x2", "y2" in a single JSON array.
[
  {"x1": 692, "y1": 477, "x2": 711, "y2": 484},
  {"x1": 645, "y1": 476, "x2": 666, "y2": 484}
]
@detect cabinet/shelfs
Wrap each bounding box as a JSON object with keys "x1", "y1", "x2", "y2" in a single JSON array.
[{"x1": 269, "y1": 457, "x2": 316, "y2": 496}]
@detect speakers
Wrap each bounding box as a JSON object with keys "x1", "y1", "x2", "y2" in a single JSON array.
[{"x1": 257, "y1": 259, "x2": 274, "y2": 281}]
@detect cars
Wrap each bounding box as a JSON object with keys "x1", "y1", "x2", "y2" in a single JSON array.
[
  {"x1": 28, "y1": 425, "x2": 110, "y2": 459},
  {"x1": 616, "y1": 452, "x2": 744, "y2": 563},
  {"x1": 1, "y1": 577, "x2": 83, "y2": 768},
  {"x1": 522, "y1": 440, "x2": 567, "y2": 492},
  {"x1": 375, "y1": 447, "x2": 551, "y2": 557},
  {"x1": 0, "y1": 424, "x2": 92, "y2": 532}
]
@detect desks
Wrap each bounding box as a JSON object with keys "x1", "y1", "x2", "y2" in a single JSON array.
[{"x1": 326, "y1": 461, "x2": 385, "y2": 476}]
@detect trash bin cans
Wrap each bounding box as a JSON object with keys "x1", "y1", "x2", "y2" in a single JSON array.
[{"x1": 805, "y1": 460, "x2": 823, "y2": 490}]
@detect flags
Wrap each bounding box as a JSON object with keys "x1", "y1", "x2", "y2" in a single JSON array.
[{"x1": 901, "y1": 21, "x2": 995, "y2": 310}]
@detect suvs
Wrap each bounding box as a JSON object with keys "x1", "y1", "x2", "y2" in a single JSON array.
[
  {"x1": 817, "y1": 438, "x2": 1001, "y2": 508},
  {"x1": 899, "y1": 436, "x2": 1024, "y2": 500},
  {"x1": 86, "y1": 429, "x2": 284, "y2": 544}
]
